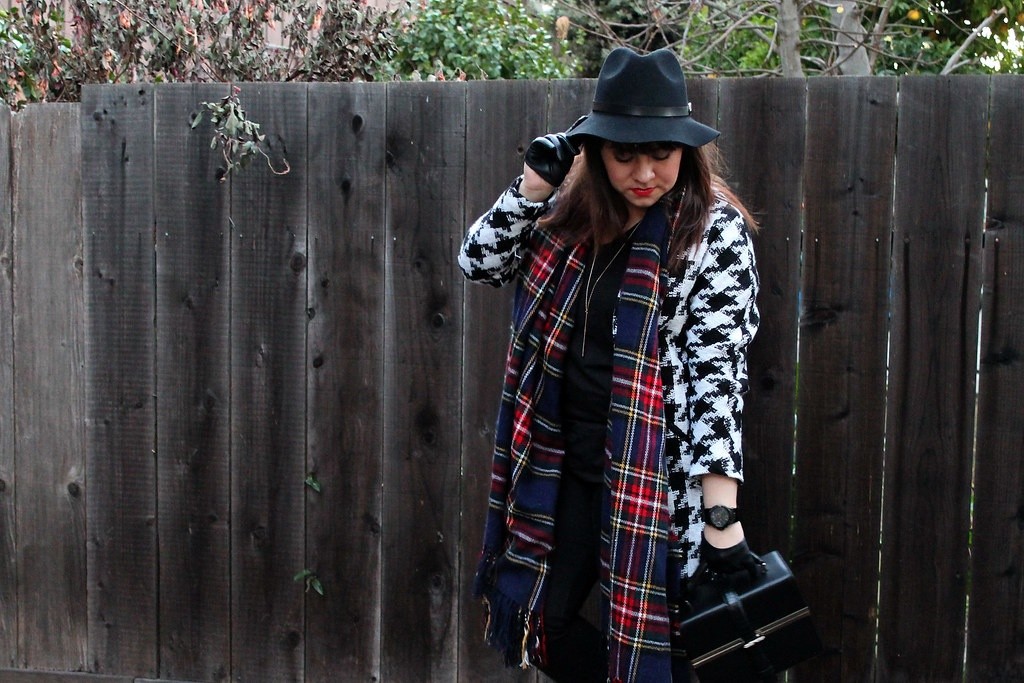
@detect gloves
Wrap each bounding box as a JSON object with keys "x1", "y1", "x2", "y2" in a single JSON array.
[
  {"x1": 524, "y1": 115, "x2": 588, "y2": 189},
  {"x1": 706, "y1": 538, "x2": 770, "y2": 593}
]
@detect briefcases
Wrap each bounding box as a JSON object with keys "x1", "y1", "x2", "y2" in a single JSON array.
[{"x1": 672, "y1": 550, "x2": 816, "y2": 683}]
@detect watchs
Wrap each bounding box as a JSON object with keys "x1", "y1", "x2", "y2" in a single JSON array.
[{"x1": 700, "y1": 505, "x2": 740, "y2": 531}]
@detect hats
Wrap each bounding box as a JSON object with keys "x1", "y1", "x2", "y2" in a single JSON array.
[{"x1": 567, "y1": 47, "x2": 722, "y2": 148}]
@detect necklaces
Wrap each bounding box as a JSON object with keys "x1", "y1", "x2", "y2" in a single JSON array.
[{"x1": 582, "y1": 216, "x2": 642, "y2": 356}]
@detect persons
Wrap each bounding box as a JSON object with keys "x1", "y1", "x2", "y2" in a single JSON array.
[{"x1": 458, "y1": 47, "x2": 769, "y2": 683}]
